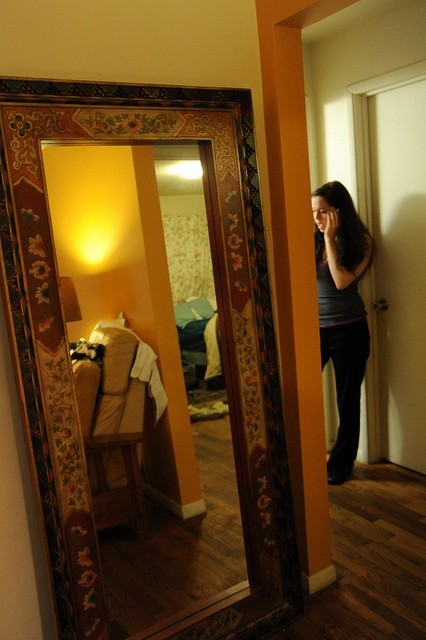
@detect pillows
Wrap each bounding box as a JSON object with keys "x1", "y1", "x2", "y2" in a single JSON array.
[
  {"x1": 189, "y1": 297, "x2": 215, "y2": 321},
  {"x1": 184, "y1": 297, "x2": 218, "y2": 320},
  {"x1": 174, "y1": 302, "x2": 195, "y2": 330}
]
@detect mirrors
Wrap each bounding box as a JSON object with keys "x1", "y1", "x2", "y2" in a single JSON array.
[{"x1": 0, "y1": 77, "x2": 303, "y2": 639}]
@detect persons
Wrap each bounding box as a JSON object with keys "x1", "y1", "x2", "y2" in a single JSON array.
[{"x1": 311, "y1": 180, "x2": 374, "y2": 486}]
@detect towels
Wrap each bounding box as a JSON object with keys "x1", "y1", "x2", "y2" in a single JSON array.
[{"x1": 129, "y1": 335, "x2": 168, "y2": 426}]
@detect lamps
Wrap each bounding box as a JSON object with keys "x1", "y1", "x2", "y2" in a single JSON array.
[{"x1": 58, "y1": 275, "x2": 82, "y2": 323}]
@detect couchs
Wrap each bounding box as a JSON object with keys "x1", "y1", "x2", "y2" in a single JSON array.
[{"x1": 62, "y1": 320, "x2": 148, "y2": 542}]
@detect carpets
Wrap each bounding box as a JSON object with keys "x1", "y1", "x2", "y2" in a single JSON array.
[{"x1": 186, "y1": 389, "x2": 230, "y2": 419}]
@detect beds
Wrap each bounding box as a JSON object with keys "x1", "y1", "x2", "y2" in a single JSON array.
[{"x1": 172, "y1": 295, "x2": 223, "y2": 381}]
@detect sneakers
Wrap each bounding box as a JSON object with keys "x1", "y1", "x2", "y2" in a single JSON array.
[{"x1": 328, "y1": 456, "x2": 354, "y2": 485}]
